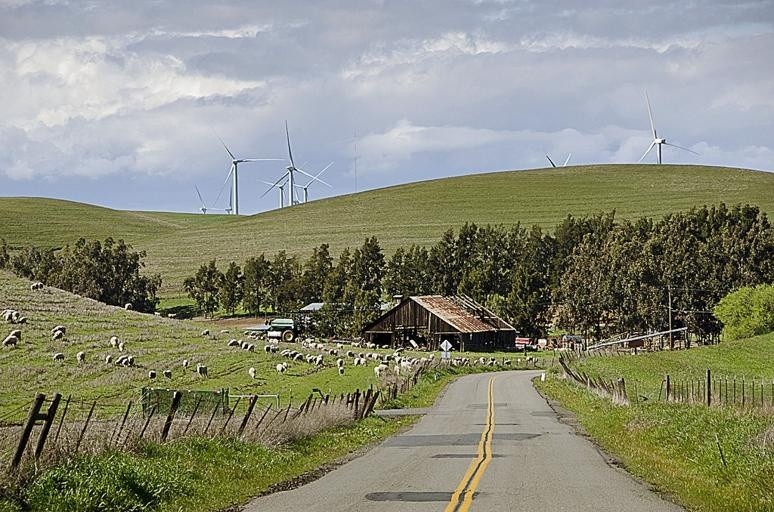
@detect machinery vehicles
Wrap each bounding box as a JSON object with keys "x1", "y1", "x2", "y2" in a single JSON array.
[{"x1": 264, "y1": 315, "x2": 299, "y2": 343}]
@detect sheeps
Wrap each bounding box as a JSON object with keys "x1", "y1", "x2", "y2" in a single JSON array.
[
  {"x1": 105, "y1": 355, "x2": 113, "y2": 364},
  {"x1": 195, "y1": 362, "x2": 207, "y2": 378},
  {"x1": 30, "y1": 282, "x2": 45, "y2": 291},
  {"x1": 118, "y1": 342, "x2": 125, "y2": 351},
  {"x1": 227, "y1": 336, "x2": 394, "y2": 378},
  {"x1": 154, "y1": 312, "x2": 160, "y2": 317},
  {"x1": 162, "y1": 370, "x2": 172, "y2": 378},
  {"x1": 182, "y1": 360, "x2": 189, "y2": 368},
  {"x1": 50, "y1": 325, "x2": 67, "y2": 341},
  {"x1": 148, "y1": 370, "x2": 157, "y2": 378},
  {"x1": 109, "y1": 337, "x2": 118, "y2": 348},
  {"x1": 75, "y1": 352, "x2": 86, "y2": 362},
  {"x1": 219, "y1": 329, "x2": 229, "y2": 333},
  {"x1": 1, "y1": 336, "x2": 17, "y2": 348},
  {"x1": 395, "y1": 348, "x2": 403, "y2": 352},
  {"x1": 124, "y1": 303, "x2": 132, "y2": 311},
  {"x1": 200, "y1": 329, "x2": 209, "y2": 334},
  {"x1": 112, "y1": 354, "x2": 135, "y2": 367},
  {"x1": 52, "y1": 352, "x2": 65, "y2": 362},
  {"x1": 5, "y1": 330, "x2": 21, "y2": 341},
  {"x1": 394, "y1": 352, "x2": 540, "y2": 370},
  {"x1": 0, "y1": 308, "x2": 29, "y2": 326},
  {"x1": 168, "y1": 313, "x2": 177, "y2": 318}
]
